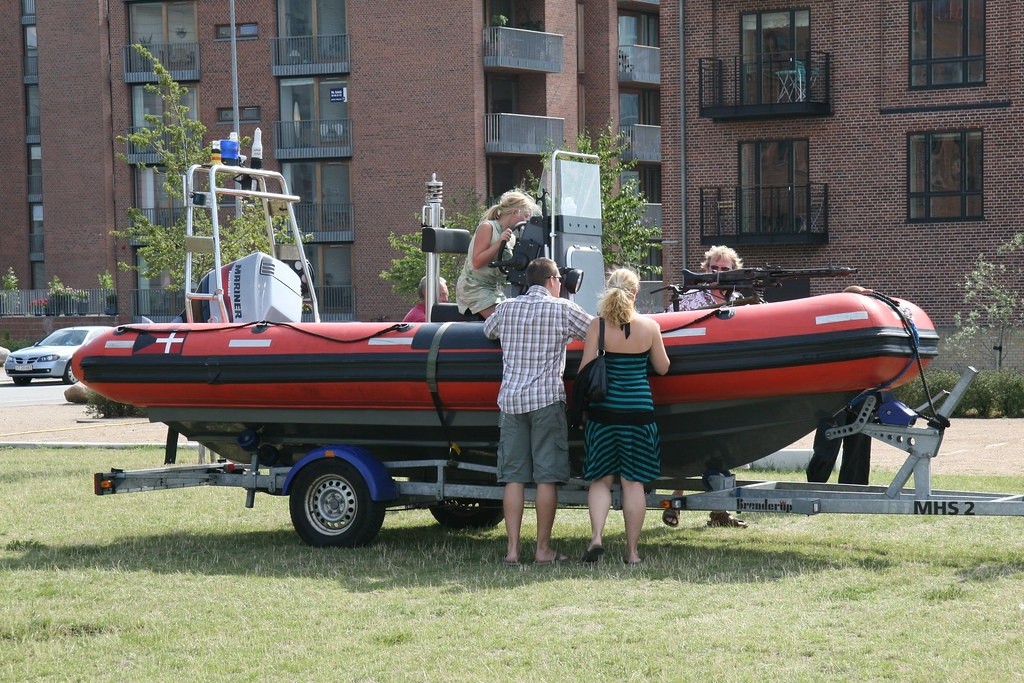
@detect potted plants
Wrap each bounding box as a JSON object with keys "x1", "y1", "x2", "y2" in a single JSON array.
[{"x1": 47, "y1": 285, "x2": 90, "y2": 317}]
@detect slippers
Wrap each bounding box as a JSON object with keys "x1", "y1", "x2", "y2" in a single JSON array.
[
  {"x1": 622, "y1": 558, "x2": 640, "y2": 565},
  {"x1": 581, "y1": 545, "x2": 605, "y2": 563},
  {"x1": 503, "y1": 554, "x2": 521, "y2": 565},
  {"x1": 533, "y1": 552, "x2": 569, "y2": 565}
]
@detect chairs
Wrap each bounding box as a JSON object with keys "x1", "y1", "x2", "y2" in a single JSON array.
[
  {"x1": 809, "y1": 65, "x2": 821, "y2": 89},
  {"x1": 421, "y1": 225, "x2": 484, "y2": 322},
  {"x1": 794, "y1": 59, "x2": 807, "y2": 102},
  {"x1": 717, "y1": 200, "x2": 735, "y2": 235}
]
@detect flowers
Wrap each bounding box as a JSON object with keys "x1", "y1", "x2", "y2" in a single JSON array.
[{"x1": 31, "y1": 299, "x2": 48, "y2": 308}]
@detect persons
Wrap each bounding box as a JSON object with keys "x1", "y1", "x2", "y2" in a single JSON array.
[
  {"x1": 806, "y1": 285, "x2": 871, "y2": 486},
  {"x1": 481, "y1": 258, "x2": 594, "y2": 565},
  {"x1": 455, "y1": 193, "x2": 536, "y2": 320},
  {"x1": 576, "y1": 269, "x2": 671, "y2": 564},
  {"x1": 661, "y1": 245, "x2": 750, "y2": 530},
  {"x1": 402, "y1": 275, "x2": 450, "y2": 322}
]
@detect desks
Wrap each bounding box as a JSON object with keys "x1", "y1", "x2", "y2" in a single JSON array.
[{"x1": 773, "y1": 70, "x2": 801, "y2": 103}]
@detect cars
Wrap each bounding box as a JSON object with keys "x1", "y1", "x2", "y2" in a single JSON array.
[{"x1": 4, "y1": 325, "x2": 113, "y2": 384}]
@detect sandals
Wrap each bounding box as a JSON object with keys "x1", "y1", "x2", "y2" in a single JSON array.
[
  {"x1": 663, "y1": 507, "x2": 681, "y2": 526},
  {"x1": 706, "y1": 511, "x2": 748, "y2": 529}
]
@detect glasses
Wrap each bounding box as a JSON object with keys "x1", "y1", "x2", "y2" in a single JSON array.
[
  {"x1": 708, "y1": 264, "x2": 730, "y2": 272},
  {"x1": 545, "y1": 275, "x2": 562, "y2": 283}
]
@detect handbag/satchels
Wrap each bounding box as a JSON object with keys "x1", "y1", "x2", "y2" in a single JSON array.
[{"x1": 576, "y1": 316, "x2": 608, "y2": 402}]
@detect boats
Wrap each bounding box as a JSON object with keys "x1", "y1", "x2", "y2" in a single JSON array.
[{"x1": 69, "y1": 127, "x2": 941, "y2": 487}]
